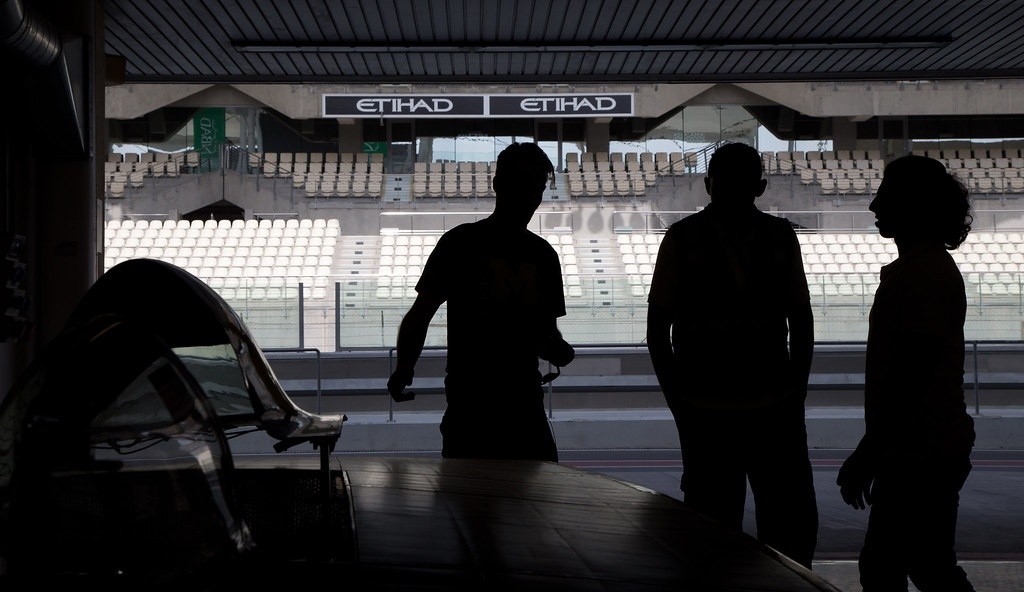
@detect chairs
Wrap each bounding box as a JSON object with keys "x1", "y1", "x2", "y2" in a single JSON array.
[{"x1": 104, "y1": 142, "x2": 1024, "y2": 303}]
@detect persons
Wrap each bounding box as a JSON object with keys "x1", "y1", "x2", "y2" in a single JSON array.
[
  {"x1": 837, "y1": 156, "x2": 977, "y2": 592},
  {"x1": 386, "y1": 142, "x2": 575, "y2": 464},
  {"x1": 646, "y1": 142, "x2": 820, "y2": 569}
]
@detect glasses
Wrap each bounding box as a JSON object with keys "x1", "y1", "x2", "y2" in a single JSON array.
[{"x1": 541, "y1": 366, "x2": 561, "y2": 384}]
